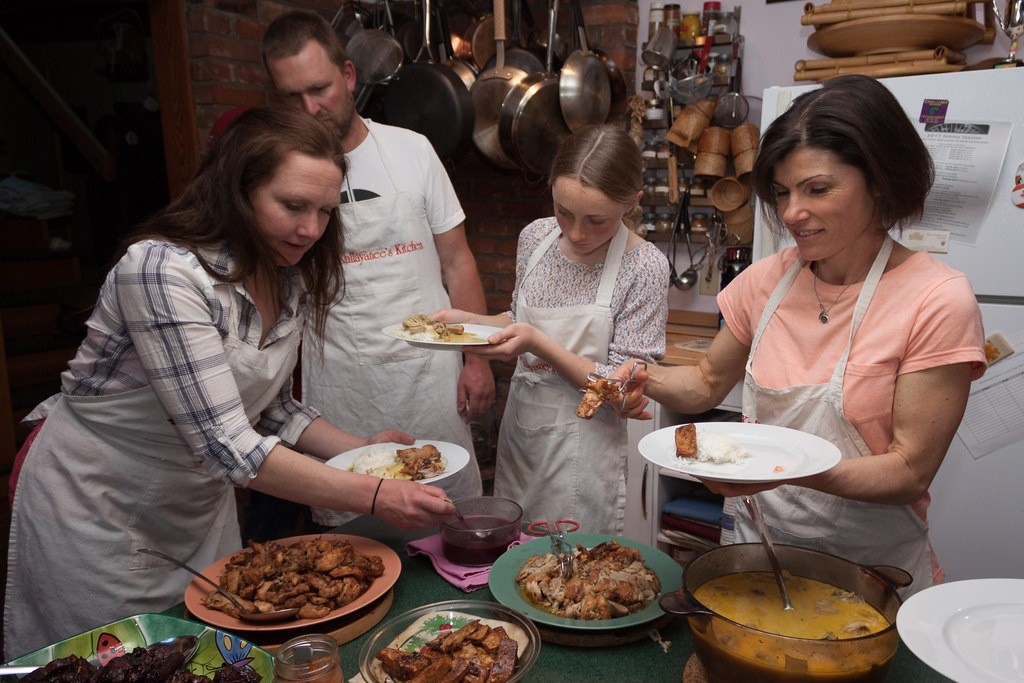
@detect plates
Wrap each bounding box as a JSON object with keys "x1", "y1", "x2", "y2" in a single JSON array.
[
  {"x1": 185, "y1": 533, "x2": 403, "y2": 632},
  {"x1": 358, "y1": 599, "x2": 542, "y2": 683},
  {"x1": 326, "y1": 440, "x2": 469, "y2": 483},
  {"x1": 1, "y1": 612, "x2": 273, "y2": 683},
  {"x1": 378, "y1": 324, "x2": 508, "y2": 350},
  {"x1": 894, "y1": 578, "x2": 1024, "y2": 683},
  {"x1": 488, "y1": 531, "x2": 684, "y2": 628},
  {"x1": 637, "y1": 422, "x2": 842, "y2": 483}
]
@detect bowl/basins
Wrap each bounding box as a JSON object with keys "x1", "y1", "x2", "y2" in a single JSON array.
[{"x1": 439, "y1": 495, "x2": 523, "y2": 567}]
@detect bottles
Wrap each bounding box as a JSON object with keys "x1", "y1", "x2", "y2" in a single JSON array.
[
  {"x1": 274, "y1": 633, "x2": 345, "y2": 683},
  {"x1": 640, "y1": 2, "x2": 738, "y2": 235}
]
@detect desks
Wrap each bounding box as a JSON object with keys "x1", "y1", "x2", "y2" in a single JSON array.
[{"x1": 158, "y1": 512, "x2": 956, "y2": 683}]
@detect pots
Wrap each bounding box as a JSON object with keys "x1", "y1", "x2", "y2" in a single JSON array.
[
  {"x1": 328, "y1": 0, "x2": 618, "y2": 187},
  {"x1": 659, "y1": 543, "x2": 912, "y2": 682}
]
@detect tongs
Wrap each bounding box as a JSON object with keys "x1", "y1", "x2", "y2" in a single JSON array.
[
  {"x1": 526, "y1": 519, "x2": 580, "y2": 578},
  {"x1": 577, "y1": 361, "x2": 648, "y2": 417}
]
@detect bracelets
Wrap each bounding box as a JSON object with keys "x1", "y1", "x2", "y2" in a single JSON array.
[{"x1": 370, "y1": 478, "x2": 384, "y2": 515}]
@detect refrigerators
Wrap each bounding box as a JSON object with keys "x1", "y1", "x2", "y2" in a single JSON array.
[{"x1": 732, "y1": 67, "x2": 1024, "y2": 583}]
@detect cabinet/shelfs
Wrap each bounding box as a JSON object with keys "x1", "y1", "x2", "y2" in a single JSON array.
[{"x1": 0, "y1": 217, "x2": 82, "y2": 476}]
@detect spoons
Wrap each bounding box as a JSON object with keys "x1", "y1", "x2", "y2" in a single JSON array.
[
  {"x1": 138, "y1": 546, "x2": 299, "y2": 623},
  {"x1": 444, "y1": 500, "x2": 496, "y2": 540}
]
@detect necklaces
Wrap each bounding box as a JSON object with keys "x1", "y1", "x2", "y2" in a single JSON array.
[{"x1": 814, "y1": 233, "x2": 885, "y2": 324}]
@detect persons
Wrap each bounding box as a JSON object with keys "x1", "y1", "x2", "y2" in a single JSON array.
[
  {"x1": 605, "y1": 74, "x2": 987, "y2": 605},
  {"x1": 424, "y1": 124, "x2": 671, "y2": 549},
  {"x1": 262, "y1": 10, "x2": 496, "y2": 533},
  {"x1": 0, "y1": 104, "x2": 455, "y2": 664}
]
